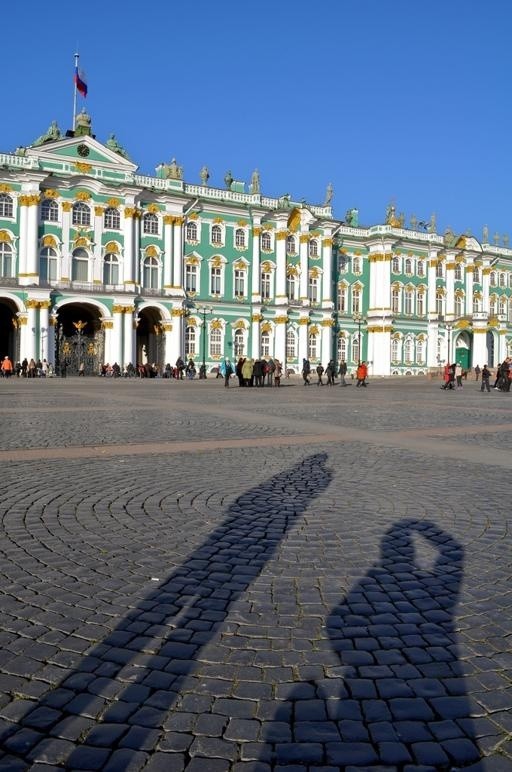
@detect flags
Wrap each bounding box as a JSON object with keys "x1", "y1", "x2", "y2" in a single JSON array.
[{"x1": 73, "y1": 64, "x2": 89, "y2": 101}]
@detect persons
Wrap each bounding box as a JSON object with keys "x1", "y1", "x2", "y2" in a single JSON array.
[
  {"x1": 199, "y1": 365, "x2": 207, "y2": 379},
  {"x1": 79, "y1": 362, "x2": 85, "y2": 376},
  {"x1": 1, "y1": 355, "x2": 69, "y2": 378},
  {"x1": 165, "y1": 355, "x2": 196, "y2": 380},
  {"x1": 325, "y1": 360, "x2": 338, "y2": 386},
  {"x1": 221, "y1": 357, "x2": 282, "y2": 387},
  {"x1": 316, "y1": 363, "x2": 324, "y2": 386},
  {"x1": 356, "y1": 360, "x2": 372, "y2": 387},
  {"x1": 301, "y1": 358, "x2": 311, "y2": 386},
  {"x1": 338, "y1": 360, "x2": 347, "y2": 384},
  {"x1": 439, "y1": 355, "x2": 512, "y2": 394},
  {"x1": 216, "y1": 363, "x2": 223, "y2": 378},
  {"x1": 350, "y1": 368, "x2": 356, "y2": 380},
  {"x1": 99, "y1": 361, "x2": 158, "y2": 379}
]
